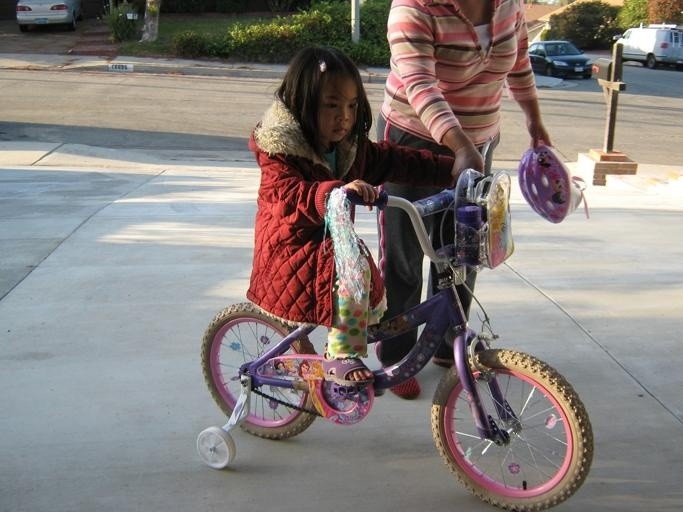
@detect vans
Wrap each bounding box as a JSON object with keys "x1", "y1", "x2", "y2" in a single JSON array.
[{"x1": 613, "y1": 23, "x2": 682, "y2": 68}]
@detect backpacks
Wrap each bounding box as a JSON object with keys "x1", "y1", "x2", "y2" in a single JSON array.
[{"x1": 453, "y1": 168, "x2": 515, "y2": 272}]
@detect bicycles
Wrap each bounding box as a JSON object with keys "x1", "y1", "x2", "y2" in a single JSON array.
[{"x1": 196, "y1": 139, "x2": 593, "y2": 512}]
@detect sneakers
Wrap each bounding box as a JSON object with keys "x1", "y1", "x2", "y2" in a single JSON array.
[{"x1": 382, "y1": 365, "x2": 420, "y2": 399}]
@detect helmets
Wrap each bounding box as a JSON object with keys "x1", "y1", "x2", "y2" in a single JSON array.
[{"x1": 518, "y1": 144, "x2": 583, "y2": 224}]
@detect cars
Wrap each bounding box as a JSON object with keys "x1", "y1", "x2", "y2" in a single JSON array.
[
  {"x1": 528, "y1": 41, "x2": 594, "y2": 78},
  {"x1": 16, "y1": 0, "x2": 84, "y2": 32}
]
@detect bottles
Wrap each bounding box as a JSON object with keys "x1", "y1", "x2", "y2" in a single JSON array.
[{"x1": 455, "y1": 206, "x2": 484, "y2": 268}]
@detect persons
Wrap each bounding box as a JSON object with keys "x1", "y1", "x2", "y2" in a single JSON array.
[
  {"x1": 375, "y1": 0, "x2": 549, "y2": 400},
  {"x1": 249, "y1": 45, "x2": 455, "y2": 389}
]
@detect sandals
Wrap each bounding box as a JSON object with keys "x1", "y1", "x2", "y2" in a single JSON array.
[{"x1": 322, "y1": 352, "x2": 375, "y2": 388}]
